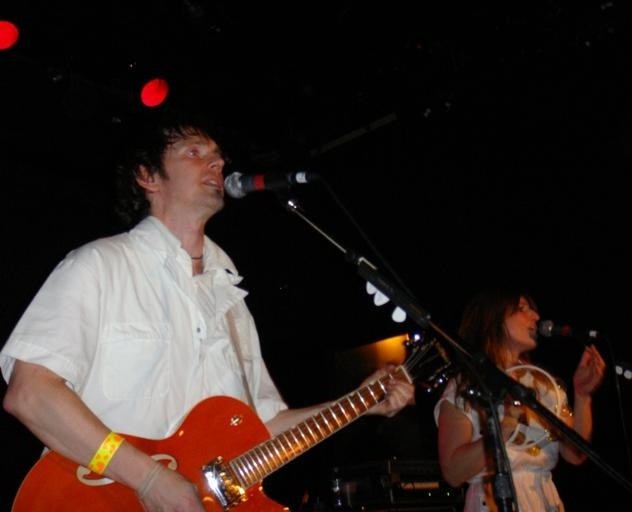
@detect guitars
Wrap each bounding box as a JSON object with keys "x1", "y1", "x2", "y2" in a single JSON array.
[{"x1": 10, "y1": 336, "x2": 452, "y2": 511}]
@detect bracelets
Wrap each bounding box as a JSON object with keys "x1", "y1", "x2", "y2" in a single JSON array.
[
  {"x1": 130, "y1": 463, "x2": 163, "y2": 497},
  {"x1": 88, "y1": 432, "x2": 125, "y2": 475}
]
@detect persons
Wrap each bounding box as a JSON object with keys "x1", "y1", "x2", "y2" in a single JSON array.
[
  {"x1": 0, "y1": 109, "x2": 415, "y2": 512},
  {"x1": 434, "y1": 289, "x2": 605, "y2": 512}
]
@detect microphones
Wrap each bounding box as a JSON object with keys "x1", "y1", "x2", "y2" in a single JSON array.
[
  {"x1": 539, "y1": 317, "x2": 600, "y2": 344},
  {"x1": 221, "y1": 168, "x2": 307, "y2": 200}
]
